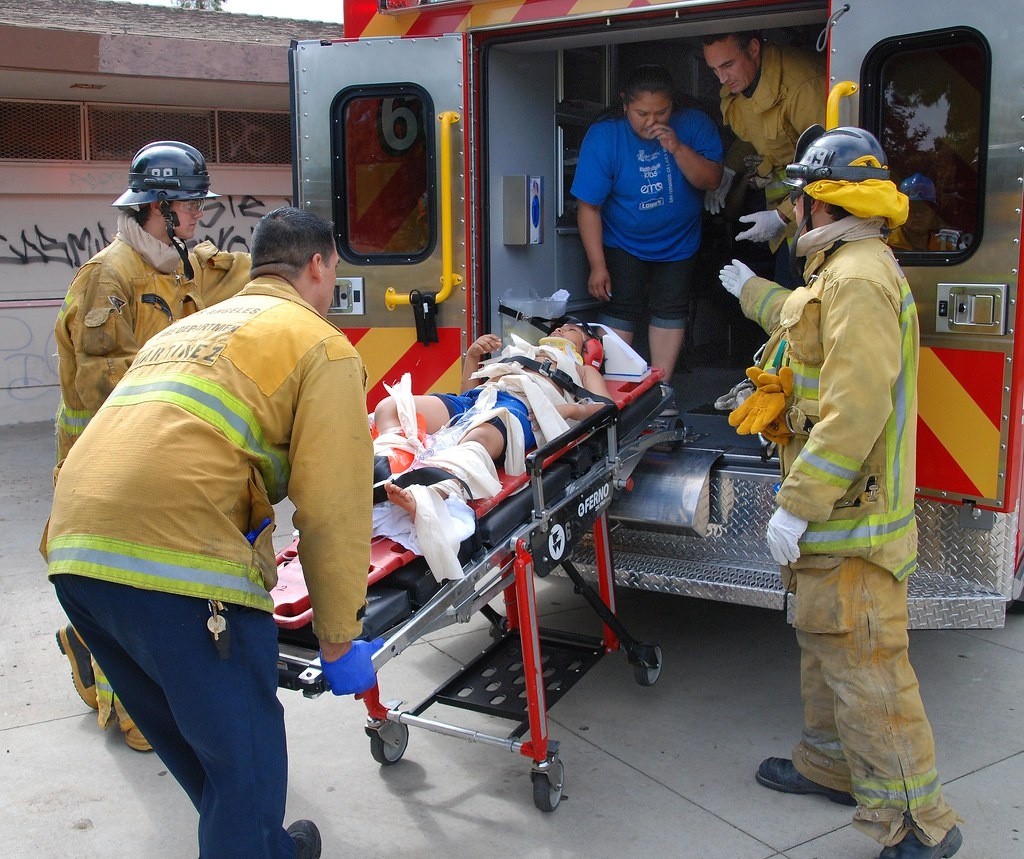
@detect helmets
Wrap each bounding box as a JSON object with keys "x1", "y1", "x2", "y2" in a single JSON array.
[
  {"x1": 899, "y1": 172, "x2": 937, "y2": 203},
  {"x1": 111, "y1": 141, "x2": 222, "y2": 206},
  {"x1": 780, "y1": 124, "x2": 891, "y2": 210}
]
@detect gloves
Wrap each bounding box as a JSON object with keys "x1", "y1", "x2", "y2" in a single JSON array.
[
  {"x1": 703, "y1": 166, "x2": 736, "y2": 215},
  {"x1": 719, "y1": 259, "x2": 756, "y2": 298},
  {"x1": 766, "y1": 506, "x2": 811, "y2": 566},
  {"x1": 735, "y1": 209, "x2": 787, "y2": 243},
  {"x1": 319, "y1": 636, "x2": 385, "y2": 697},
  {"x1": 728, "y1": 366, "x2": 795, "y2": 445}
]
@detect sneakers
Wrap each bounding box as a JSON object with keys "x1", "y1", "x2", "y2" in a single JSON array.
[
  {"x1": 116, "y1": 695, "x2": 154, "y2": 751},
  {"x1": 55, "y1": 622, "x2": 99, "y2": 709},
  {"x1": 285, "y1": 820, "x2": 322, "y2": 859},
  {"x1": 657, "y1": 384, "x2": 678, "y2": 417}
]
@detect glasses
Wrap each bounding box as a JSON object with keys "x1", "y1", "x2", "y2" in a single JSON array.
[
  {"x1": 173, "y1": 200, "x2": 205, "y2": 214},
  {"x1": 790, "y1": 189, "x2": 805, "y2": 206}
]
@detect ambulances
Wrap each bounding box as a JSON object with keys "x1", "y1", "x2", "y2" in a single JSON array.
[{"x1": 288, "y1": 0, "x2": 1022, "y2": 630}]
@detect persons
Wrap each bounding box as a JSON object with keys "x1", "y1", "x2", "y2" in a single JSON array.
[
  {"x1": 718, "y1": 126, "x2": 964, "y2": 859},
  {"x1": 39, "y1": 206, "x2": 385, "y2": 859},
  {"x1": 376, "y1": 315, "x2": 615, "y2": 521},
  {"x1": 53, "y1": 142, "x2": 252, "y2": 753},
  {"x1": 883, "y1": 173, "x2": 957, "y2": 256},
  {"x1": 570, "y1": 54, "x2": 723, "y2": 416},
  {"x1": 703, "y1": 29, "x2": 825, "y2": 291}
]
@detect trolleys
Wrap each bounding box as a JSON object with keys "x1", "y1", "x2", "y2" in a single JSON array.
[{"x1": 272, "y1": 320, "x2": 680, "y2": 812}]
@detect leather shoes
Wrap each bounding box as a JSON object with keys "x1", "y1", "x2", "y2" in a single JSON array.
[
  {"x1": 755, "y1": 756, "x2": 857, "y2": 806},
  {"x1": 880, "y1": 824, "x2": 963, "y2": 859}
]
